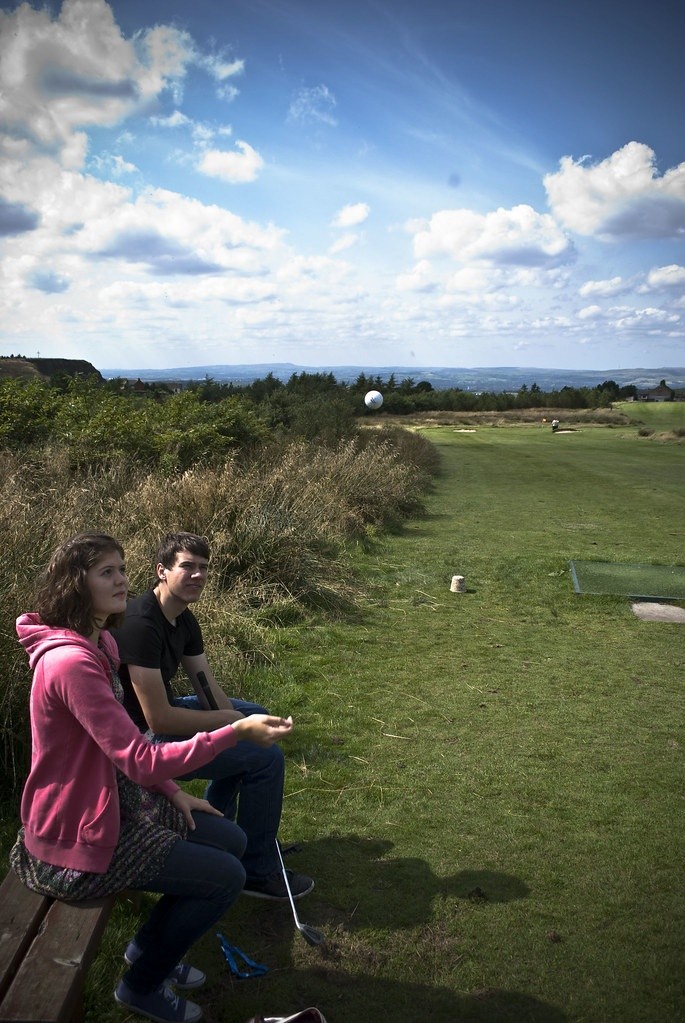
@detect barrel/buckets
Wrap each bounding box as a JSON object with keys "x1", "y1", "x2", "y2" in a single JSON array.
[{"x1": 450, "y1": 575, "x2": 467, "y2": 593}]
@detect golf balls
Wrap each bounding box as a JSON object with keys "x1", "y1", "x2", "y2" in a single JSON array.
[{"x1": 364, "y1": 390, "x2": 384, "y2": 410}]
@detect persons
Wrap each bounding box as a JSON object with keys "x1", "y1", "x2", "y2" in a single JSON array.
[
  {"x1": 104, "y1": 531, "x2": 314, "y2": 901},
  {"x1": 11, "y1": 532, "x2": 294, "y2": 1021}
]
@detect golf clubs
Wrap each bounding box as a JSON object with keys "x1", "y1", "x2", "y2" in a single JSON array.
[
  {"x1": 275, "y1": 836, "x2": 324, "y2": 947},
  {"x1": 195, "y1": 669, "x2": 220, "y2": 711}
]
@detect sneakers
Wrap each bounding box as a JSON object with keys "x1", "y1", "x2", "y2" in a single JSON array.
[
  {"x1": 243, "y1": 868, "x2": 315, "y2": 902},
  {"x1": 126, "y1": 942, "x2": 207, "y2": 989},
  {"x1": 113, "y1": 972, "x2": 202, "y2": 1023}
]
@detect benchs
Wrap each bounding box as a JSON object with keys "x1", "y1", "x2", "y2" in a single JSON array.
[{"x1": 0, "y1": 868, "x2": 116, "y2": 1023}]
